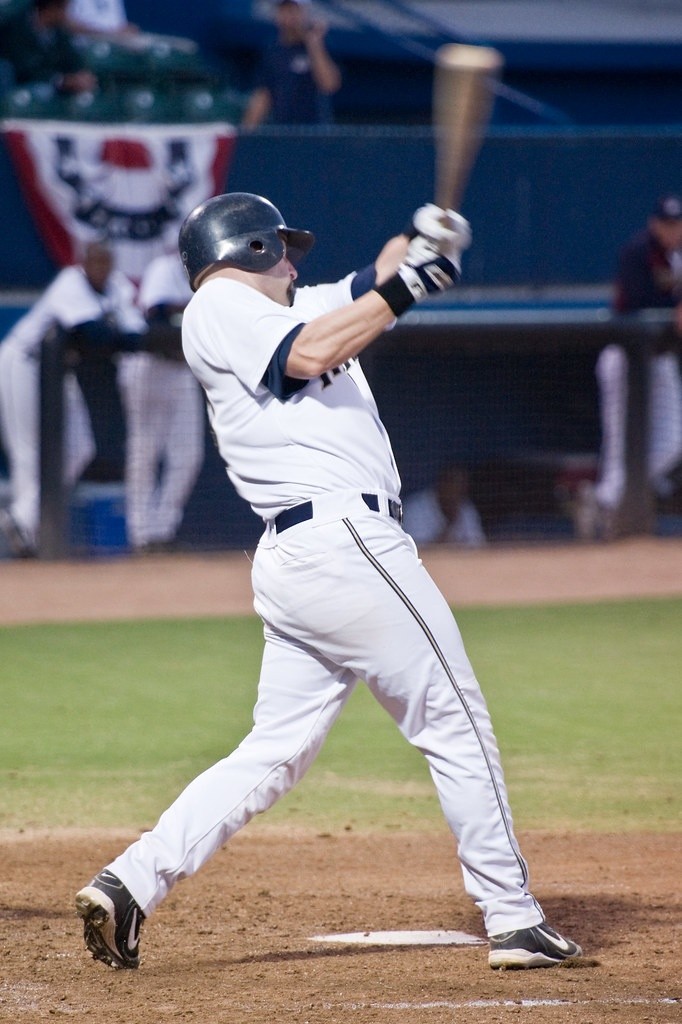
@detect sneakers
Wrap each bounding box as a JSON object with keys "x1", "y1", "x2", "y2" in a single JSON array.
[
  {"x1": 73, "y1": 866, "x2": 148, "y2": 969},
  {"x1": 486, "y1": 921, "x2": 584, "y2": 969}
]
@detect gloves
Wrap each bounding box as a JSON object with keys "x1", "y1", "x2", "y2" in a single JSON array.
[{"x1": 395, "y1": 234, "x2": 470, "y2": 304}]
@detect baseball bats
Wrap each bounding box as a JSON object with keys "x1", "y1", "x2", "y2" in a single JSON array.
[{"x1": 434, "y1": 44, "x2": 502, "y2": 253}]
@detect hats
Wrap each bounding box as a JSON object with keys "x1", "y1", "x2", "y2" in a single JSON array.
[{"x1": 647, "y1": 195, "x2": 682, "y2": 219}]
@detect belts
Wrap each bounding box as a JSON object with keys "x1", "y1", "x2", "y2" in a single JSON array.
[{"x1": 274, "y1": 490, "x2": 403, "y2": 535}]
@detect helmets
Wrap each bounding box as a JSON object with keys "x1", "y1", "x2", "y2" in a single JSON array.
[{"x1": 177, "y1": 191, "x2": 315, "y2": 292}]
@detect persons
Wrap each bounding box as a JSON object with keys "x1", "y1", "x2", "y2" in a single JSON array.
[
  {"x1": 0, "y1": 245, "x2": 205, "y2": 558},
  {"x1": 237, "y1": 0, "x2": 341, "y2": 135},
  {"x1": 0, "y1": 0, "x2": 142, "y2": 110},
  {"x1": 400, "y1": 463, "x2": 488, "y2": 548},
  {"x1": 580, "y1": 197, "x2": 682, "y2": 538},
  {"x1": 75, "y1": 192, "x2": 582, "y2": 969}
]
